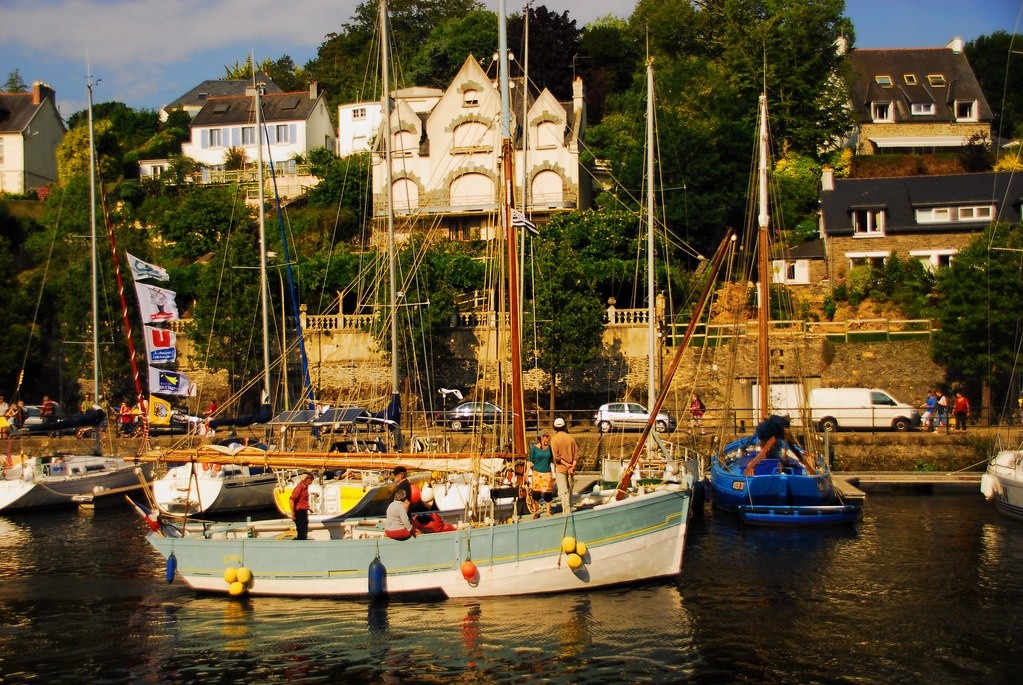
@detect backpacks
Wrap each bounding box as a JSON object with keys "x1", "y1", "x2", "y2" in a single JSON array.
[{"x1": 694, "y1": 399, "x2": 707, "y2": 413}]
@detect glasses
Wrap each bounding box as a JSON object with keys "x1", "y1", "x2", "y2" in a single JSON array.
[{"x1": 542, "y1": 434, "x2": 549, "y2": 438}]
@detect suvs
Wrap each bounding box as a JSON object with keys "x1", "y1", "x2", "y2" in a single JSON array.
[{"x1": 594, "y1": 402, "x2": 677, "y2": 433}]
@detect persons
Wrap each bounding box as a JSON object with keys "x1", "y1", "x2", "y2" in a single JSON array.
[
  {"x1": 918, "y1": 390, "x2": 936, "y2": 430},
  {"x1": 551, "y1": 418, "x2": 578, "y2": 514},
  {"x1": 523, "y1": 430, "x2": 556, "y2": 519},
  {"x1": 40, "y1": 396, "x2": 55, "y2": 438},
  {"x1": 384, "y1": 489, "x2": 418, "y2": 539},
  {"x1": 932, "y1": 390, "x2": 948, "y2": 432},
  {"x1": 99, "y1": 395, "x2": 117, "y2": 433},
  {"x1": 952, "y1": 392, "x2": 969, "y2": 431},
  {"x1": 687, "y1": 393, "x2": 705, "y2": 435},
  {"x1": 81, "y1": 394, "x2": 94, "y2": 414},
  {"x1": 757, "y1": 414, "x2": 790, "y2": 459},
  {"x1": 119, "y1": 401, "x2": 132, "y2": 431},
  {"x1": 390, "y1": 466, "x2": 411, "y2": 521},
  {"x1": 203, "y1": 398, "x2": 217, "y2": 437},
  {"x1": 290, "y1": 471, "x2": 313, "y2": 540},
  {"x1": 0, "y1": 396, "x2": 28, "y2": 439}
]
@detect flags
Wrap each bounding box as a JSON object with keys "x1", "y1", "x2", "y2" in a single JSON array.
[{"x1": 126, "y1": 251, "x2": 189, "y2": 424}]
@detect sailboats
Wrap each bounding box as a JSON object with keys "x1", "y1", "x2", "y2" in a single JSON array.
[
  {"x1": 979, "y1": 1, "x2": 1022, "y2": 522},
  {"x1": 0, "y1": 0, "x2": 702, "y2": 610},
  {"x1": 707, "y1": 33, "x2": 865, "y2": 532}
]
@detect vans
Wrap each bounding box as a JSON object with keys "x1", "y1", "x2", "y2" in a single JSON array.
[{"x1": 809, "y1": 386, "x2": 922, "y2": 433}]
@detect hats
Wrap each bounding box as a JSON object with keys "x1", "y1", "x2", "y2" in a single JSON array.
[
  {"x1": 391, "y1": 466, "x2": 407, "y2": 475},
  {"x1": 301, "y1": 473, "x2": 312, "y2": 481},
  {"x1": 553, "y1": 418, "x2": 565, "y2": 428},
  {"x1": 537, "y1": 429, "x2": 549, "y2": 437}
]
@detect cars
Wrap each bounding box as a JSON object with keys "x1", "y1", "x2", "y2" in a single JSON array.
[
  {"x1": 20, "y1": 404, "x2": 108, "y2": 438},
  {"x1": 434, "y1": 400, "x2": 537, "y2": 432}
]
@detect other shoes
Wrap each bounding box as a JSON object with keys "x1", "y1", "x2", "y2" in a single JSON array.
[
  {"x1": 699, "y1": 433, "x2": 706, "y2": 435},
  {"x1": 932, "y1": 431, "x2": 939, "y2": 433}
]
